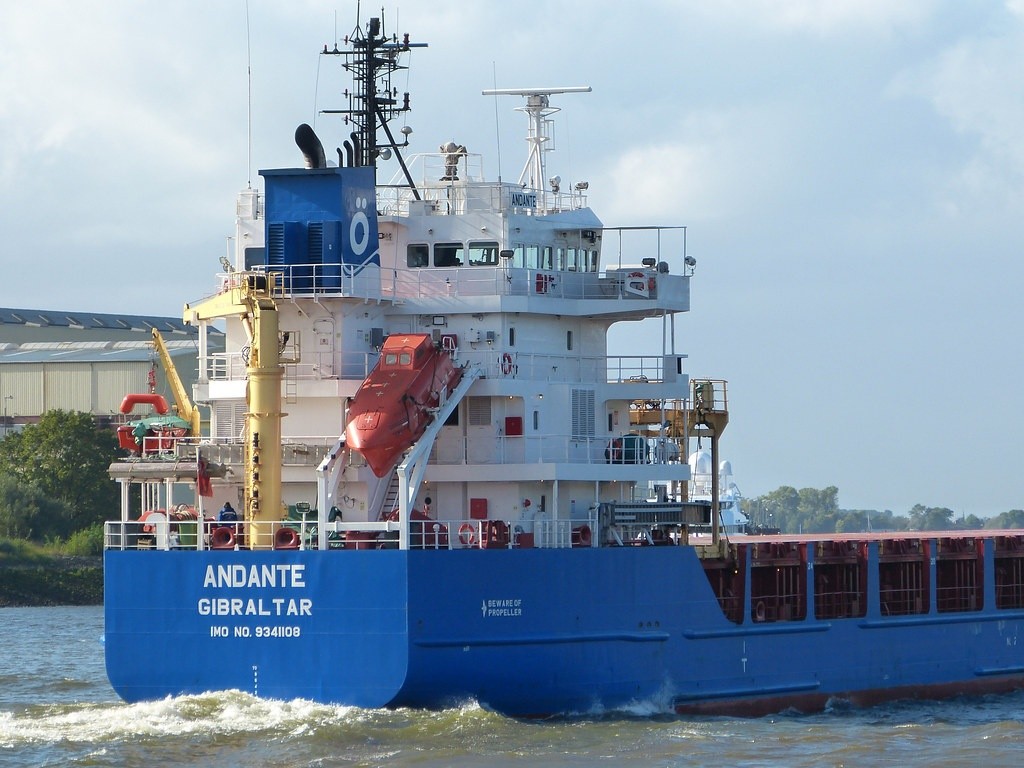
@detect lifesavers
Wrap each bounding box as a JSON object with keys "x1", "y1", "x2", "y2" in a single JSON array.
[
  {"x1": 608, "y1": 439, "x2": 621, "y2": 459},
  {"x1": 458, "y1": 524, "x2": 475, "y2": 545},
  {"x1": 499, "y1": 353, "x2": 512, "y2": 375}
]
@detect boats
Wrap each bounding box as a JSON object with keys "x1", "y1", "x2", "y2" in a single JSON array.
[
  {"x1": 100, "y1": 0, "x2": 1024, "y2": 724},
  {"x1": 672, "y1": 450, "x2": 750, "y2": 536},
  {"x1": 344, "y1": 333, "x2": 466, "y2": 480}
]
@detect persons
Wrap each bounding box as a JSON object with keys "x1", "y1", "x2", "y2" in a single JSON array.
[{"x1": 218, "y1": 501, "x2": 237, "y2": 541}]
